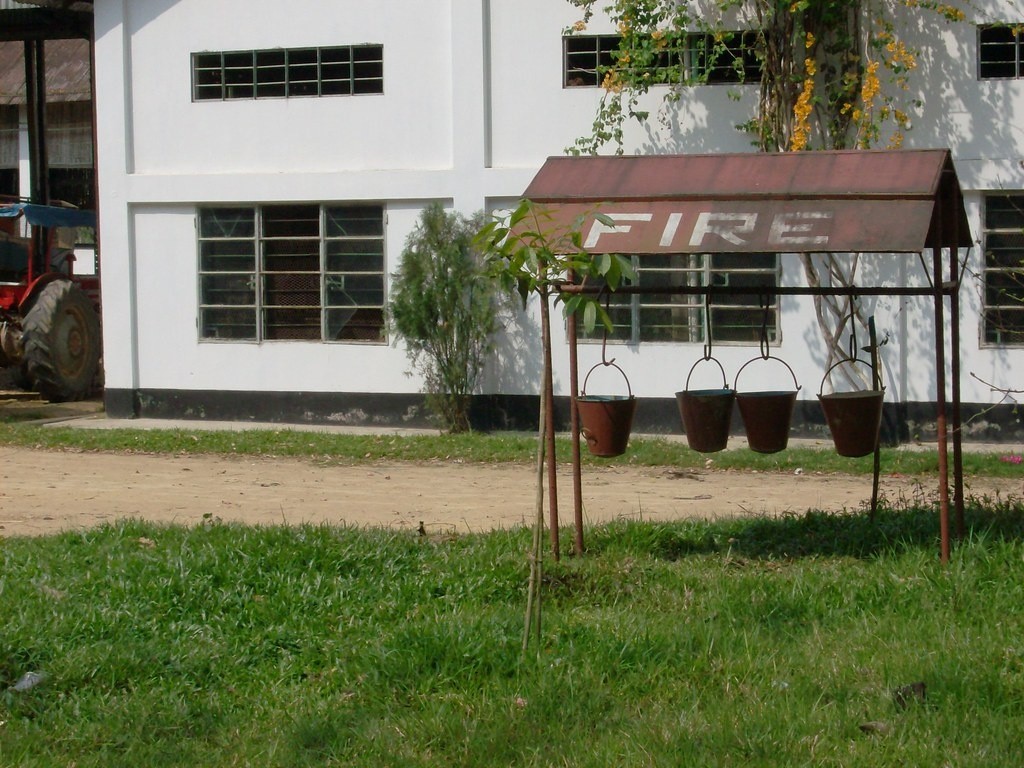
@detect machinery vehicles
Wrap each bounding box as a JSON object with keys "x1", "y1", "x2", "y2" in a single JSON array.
[{"x1": 0, "y1": 195, "x2": 104, "y2": 405}]
